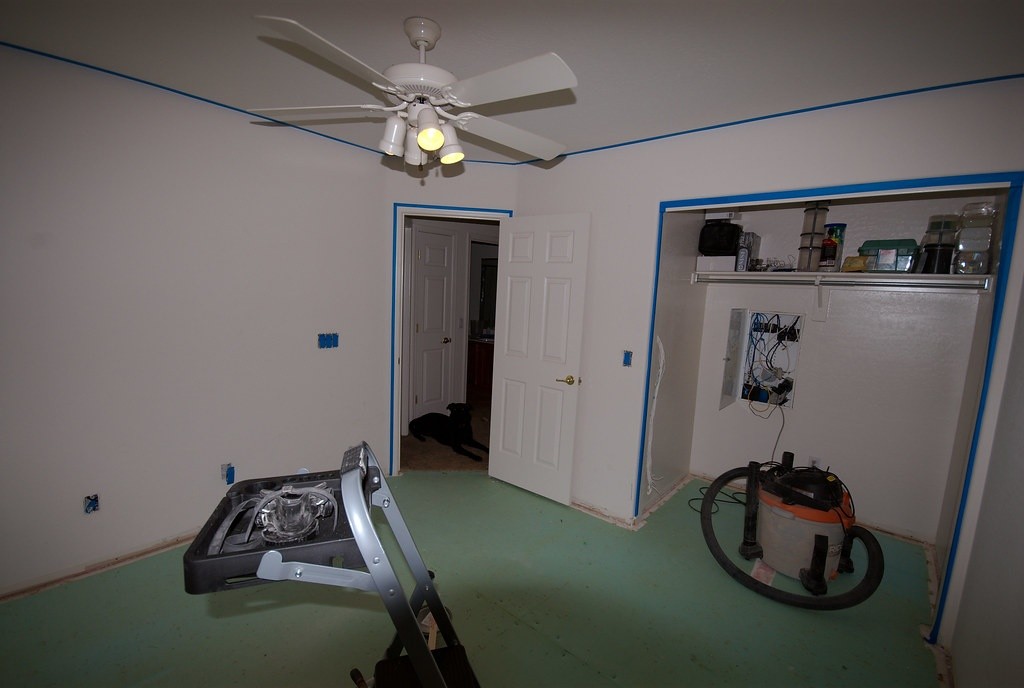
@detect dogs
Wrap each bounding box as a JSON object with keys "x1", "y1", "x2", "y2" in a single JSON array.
[{"x1": 409, "y1": 403, "x2": 490, "y2": 462}]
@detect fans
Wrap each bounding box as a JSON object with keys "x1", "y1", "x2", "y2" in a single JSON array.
[{"x1": 246, "y1": 16, "x2": 577, "y2": 168}]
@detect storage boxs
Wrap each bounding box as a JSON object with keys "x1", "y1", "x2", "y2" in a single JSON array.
[{"x1": 856, "y1": 201, "x2": 996, "y2": 275}]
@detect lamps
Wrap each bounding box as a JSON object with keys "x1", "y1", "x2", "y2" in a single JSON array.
[{"x1": 379, "y1": 98, "x2": 465, "y2": 165}]
